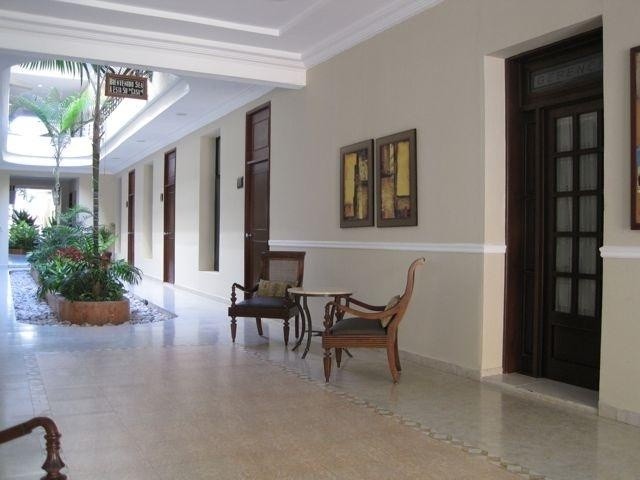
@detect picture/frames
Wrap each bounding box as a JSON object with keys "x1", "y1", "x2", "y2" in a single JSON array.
[
  {"x1": 632, "y1": 48, "x2": 640, "y2": 231},
  {"x1": 340, "y1": 138, "x2": 374, "y2": 228},
  {"x1": 374, "y1": 128, "x2": 418, "y2": 228}
]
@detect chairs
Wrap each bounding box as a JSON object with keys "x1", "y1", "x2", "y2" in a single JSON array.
[
  {"x1": 227, "y1": 250, "x2": 306, "y2": 345},
  {"x1": 0, "y1": 417, "x2": 66, "y2": 480},
  {"x1": 322, "y1": 257, "x2": 428, "y2": 382}
]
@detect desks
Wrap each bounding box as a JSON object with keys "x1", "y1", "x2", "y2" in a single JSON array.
[{"x1": 287, "y1": 285, "x2": 352, "y2": 359}]
